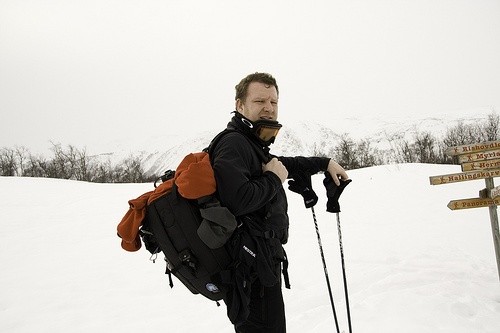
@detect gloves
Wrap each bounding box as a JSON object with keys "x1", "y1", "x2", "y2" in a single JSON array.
[
  {"x1": 323, "y1": 170, "x2": 352, "y2": 213},
  {"x1": 288, "y1": 169, "x2": 319, "y2": 209}
]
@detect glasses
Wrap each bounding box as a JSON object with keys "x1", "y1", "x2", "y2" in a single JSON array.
[{"x1": 232, "y1": 111, "x2": 282, "y2": 147}]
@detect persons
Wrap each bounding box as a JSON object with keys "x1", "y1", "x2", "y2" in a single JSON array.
[{"x1": 207, "y1": 72, "x2": 349, "y2": 333}]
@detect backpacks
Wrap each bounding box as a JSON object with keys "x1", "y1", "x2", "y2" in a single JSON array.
[{"x1": 140, "y1": 127, "x2": 270, "y2": 304}]
